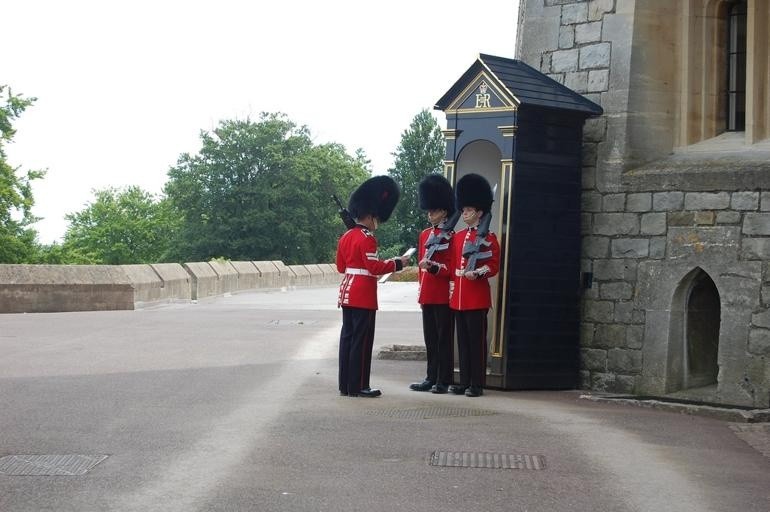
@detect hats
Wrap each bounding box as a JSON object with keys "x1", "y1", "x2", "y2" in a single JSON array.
[
  {"x1": 419, "y1": 175, "x2": 455, "y2": 219},
  {"x1": 349, "y1": 176, "x2": 399, "y2": 223},
  {"x1": 457, "y1": 174, "x2": 492, "y2": 219}
]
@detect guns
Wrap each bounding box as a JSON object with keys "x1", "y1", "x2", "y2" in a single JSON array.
[
  {"x1": 462, "y1": 212, "x2": 492, "y2": 276},
  {"x1": 331, "y1": 195, "x2": 357, "y2": 230},
  {"x1": 424, "y1": 210, "x2": 462, "y2": 259}
]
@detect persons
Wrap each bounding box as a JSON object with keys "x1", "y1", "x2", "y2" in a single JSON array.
[
  {"x1": 336, "y1": 210, "x2": 411, "y2": 398},
  {"x1": 447, "y1": 206, "x2": 501, "y2": 398},
  {"x1": 409, "y1": 207, "x2": 457, "y2": 394}
]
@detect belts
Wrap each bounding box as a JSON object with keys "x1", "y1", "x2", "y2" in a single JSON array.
[
  {"x1": 346, "y1": 268, "x2": 378, "y2": 277},
  {"x1": 421, "y1": 264, "x2": 447, "y2": 271},
  {"x1": 455, "y1": 269, "x2": 464, "y2": 276}
]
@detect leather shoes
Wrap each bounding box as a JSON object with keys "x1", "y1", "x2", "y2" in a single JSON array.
[
  {"x1": 339, "y1": 389, "x2": 347, "y2": 396},
  {"x1": 348, "y1": 388, "x2": 381, "y2": 396},
  {"x1": 410, "y1": 381, "x2": 434, "y2": 391},
  {"x1": 465, "y1": 386, "x2": 483, "y2": 396},
  {"x1": 448, "y1": 385, "x2": 468, "y2": 394},
  {"x1": 431, "y1": 384, "x2": 448, "y2": 393}
]
